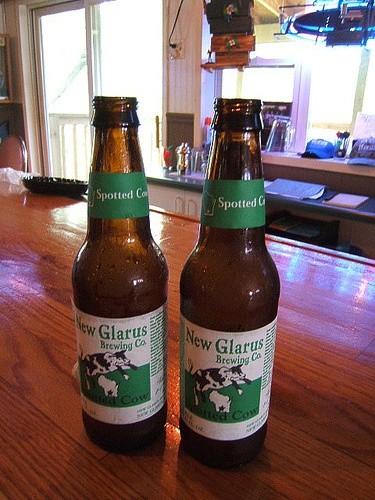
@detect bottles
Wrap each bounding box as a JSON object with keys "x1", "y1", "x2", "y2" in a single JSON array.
[
  {"x1": 179, "y1": 98, "x2": 280, "y2": 463},
  {"x1": 201, "y1": 117, "x2": 211, "y2": 149},
  {"x1": 72, "y1": 96, "x2": 165, "y2": 453}
]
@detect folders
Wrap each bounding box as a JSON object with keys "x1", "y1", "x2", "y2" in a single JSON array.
[{"x1": 265, "y1": 177, "x2": 330, "y2": 204}]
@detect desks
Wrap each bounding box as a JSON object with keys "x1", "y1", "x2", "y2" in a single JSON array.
[{"x1": 0, "y1": 182, "x2": 375, "y2": 500}]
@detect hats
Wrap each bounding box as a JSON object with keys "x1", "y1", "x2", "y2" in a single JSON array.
[
  {"x1": 301, "y1": 139, "x2": 335, "y2": 158},
  {"x1": 347, "y1": 136, "x2": 375, "y2": 166}
]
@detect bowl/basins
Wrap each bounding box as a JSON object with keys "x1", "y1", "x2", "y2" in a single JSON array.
[{"x1": 21, "y1": 176, "x2": 88, "y2": 195}]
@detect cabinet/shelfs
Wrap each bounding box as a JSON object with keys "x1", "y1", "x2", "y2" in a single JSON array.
[{"x1": 146, "y1": 174, "x2": 204, "y2": 218}]
